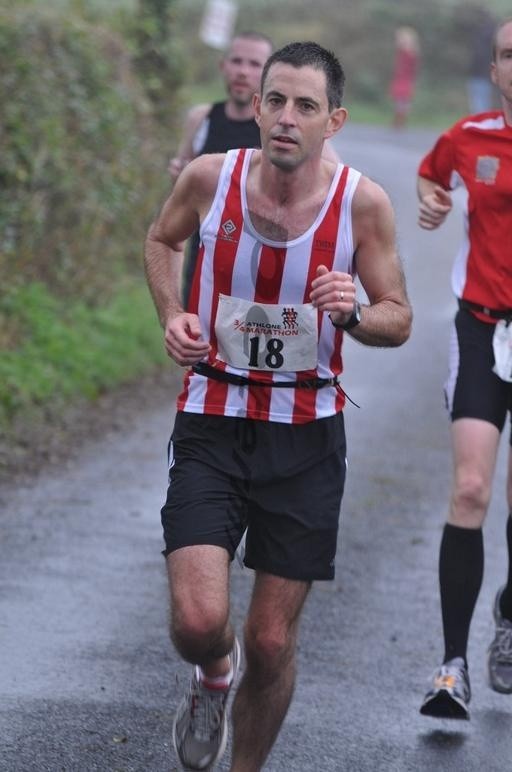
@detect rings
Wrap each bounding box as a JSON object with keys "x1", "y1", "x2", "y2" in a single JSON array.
[{"x1": 340, "y1": 291, "x2": 345, "y2": 300}]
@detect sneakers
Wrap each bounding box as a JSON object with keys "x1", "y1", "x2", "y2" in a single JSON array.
[
  {"x1": 489, "y1": 585, "x2": 512, "y2": 694},
  {"x1": 174, "y1": 638, "x2": 242, "y2": 772},
  {"x1": 419, "y1": 657, "x2": 473, "y2": 721}
]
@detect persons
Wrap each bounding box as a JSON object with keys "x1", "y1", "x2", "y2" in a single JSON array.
[
  {"x1": 458, "y1": 7, "x2": 499, "y2": 116},
  {"x1": 165, "y1": 30, "x2": 341, "y2": 312},
  {"x1": 415, "y1": 18, "x2": 512, "y2": 723},
  {"x1": 390, "y1": 25, "x2": 422, "y2": 128},
  {"x1": 144, "y1": 41, "x2": 413, "y2": 772}
]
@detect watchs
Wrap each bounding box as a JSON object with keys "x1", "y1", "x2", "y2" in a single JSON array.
[{"x1": 328, "y1": 302, "x2": 362, "y2": 331}]
